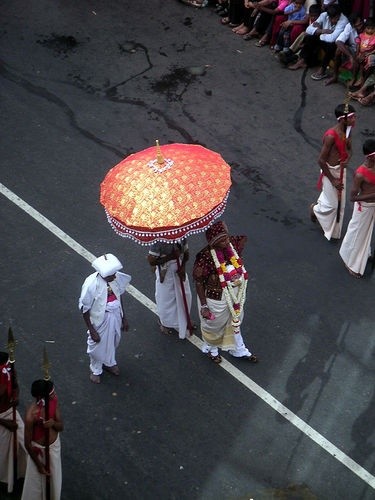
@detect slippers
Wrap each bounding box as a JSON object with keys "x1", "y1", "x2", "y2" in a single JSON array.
[
  {"x1": 358, "y1": 98, "x2": 375, "y2": 106},
  {"x1": 243, "y1": 33, "x2": 255, "y2": 40},
  {"x1": 311, "y1": 72, "x2": 327, "y2": 80},
  {"x1": 344, "y1": 91, "x2": 360, "y2": 100}
]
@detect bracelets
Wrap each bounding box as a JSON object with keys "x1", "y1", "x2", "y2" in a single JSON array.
[{"x1": 200, "y1": 304, "x2": 209, "y2": 309}]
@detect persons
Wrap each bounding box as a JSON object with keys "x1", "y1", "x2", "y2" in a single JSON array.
[
  {"x1": 147, "y1": 240, "x2": 198, "y2": 339},
  {"x1": 0, "y1": 351, "x2": 28, "y2": 493},
  {"x1": 339, "y1": 139, "x2": 375, "y2": 278},
  {"x1": 192, "y1": 221, "x2": 259, "y2": 364},
  {"x1": 311, "y1": 104, "x2": 357, "y2": 241},
  {"x1": 22, "y1": 378, "x2": 64, "y2": 500},
  {"x1": 78, "y1": 253, "x2": 132, "y2": 384},
  {"x1": 187, "y1": 0, "x2": 375, "y2": 105}
]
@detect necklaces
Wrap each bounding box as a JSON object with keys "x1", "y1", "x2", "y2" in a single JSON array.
[{"x1": 209, "y1": 242, "x2": 249, "y2": 334}]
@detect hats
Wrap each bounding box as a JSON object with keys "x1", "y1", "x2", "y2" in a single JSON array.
[{"x1": 205, "y1": 222, "x2": 230, "y2": 246}]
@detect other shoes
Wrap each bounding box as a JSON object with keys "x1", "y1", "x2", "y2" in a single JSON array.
[
  {"x1": 221, "y1": 17, "x2": 249, "y2": 35},
  {"x1": 209, "y1": 353, "x2": 222, "y2": 364},
  {"x1": 255, "y1": 38, "x2": 267, "y2": 47},
  {"x1": 244, "y1": 354, "x2": 257, "y2": 361}
]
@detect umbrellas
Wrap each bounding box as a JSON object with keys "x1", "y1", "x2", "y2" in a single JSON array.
[{"x1": 99, "y1": 139, "x2": 232, "y2": 246}]
